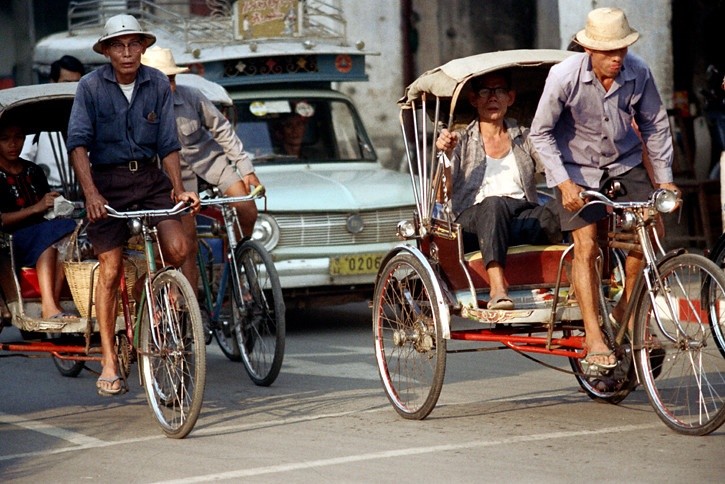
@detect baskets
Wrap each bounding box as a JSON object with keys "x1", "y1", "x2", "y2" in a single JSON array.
[{"x1": 64, "y1": 218, "x2": 148, "y2": 318}]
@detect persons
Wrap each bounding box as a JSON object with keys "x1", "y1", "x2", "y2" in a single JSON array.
[
  {"x1": 66, "y1": 14, "x2": 201, "y2": 394},
  {"x1": 435, "y1": 73, "x2": 609, "y2": 309},
  {"x1": 19, "y1": 55, "x2": 86, "y2": 194},
  {"x1": 0, "y1": 122, "x2": 77, "y2": 320},
  {"x1": 270, "y1": 112, "x2": 322, "y2": 161},
  {"x1": 529, "y1": 7, "x2": 682, "y2": 368},
  {"x1": 140, "y1": 48, "x2": 265, "y2": 328}
]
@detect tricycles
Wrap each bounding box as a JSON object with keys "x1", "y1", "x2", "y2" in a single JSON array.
[
  {"x1": 178, "y1": 182, "x2": 286, "y2": 388},
  {"x1": 0, "y1": 81, "x2": 211, "y2": 437},
  {"x1": 372, "y1": 49, "x2": 725, "y2": 442}
]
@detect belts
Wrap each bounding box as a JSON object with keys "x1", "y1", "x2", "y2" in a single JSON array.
[{"x1": 92, "y1": 156, "x2": 157, "y2": 171}]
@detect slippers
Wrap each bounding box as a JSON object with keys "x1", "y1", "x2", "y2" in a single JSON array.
[
  {"x1": 164, "y1": 293, "x2": 191, "y2": 312},
  {"x1": 486, "y1": 296, "x2": 516, "y2": 311},
  {"x1": 40, "y1": 312, "x2": 81, "y2": 323},
  {"x1": 609, "y1": 311, "x2": 656, "y2": 354},
  {"x1": 97, "y1": 375, "x2": 123, "y2": 393},
  {"x1": 240, "y1": 286, "x2": 254, "y2": 304}
]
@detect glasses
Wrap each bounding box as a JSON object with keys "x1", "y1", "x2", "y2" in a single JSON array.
[
  {"x1": 109, "y1": 41, "x2": 143, "y2": 53},
  {"x1": 477, "y1": 86, "x2": 509, "y2": 98}
]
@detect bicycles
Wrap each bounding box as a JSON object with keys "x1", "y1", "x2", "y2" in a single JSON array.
[{"x1": 703, "y1": 228, "x2": 724, "y2": 355}]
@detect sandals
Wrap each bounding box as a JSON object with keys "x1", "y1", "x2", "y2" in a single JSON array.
[{"x1": 582, "y1": 350, "x2": 618, "y2": 369}]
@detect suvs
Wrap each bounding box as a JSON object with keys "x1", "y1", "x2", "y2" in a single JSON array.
[{"x1": 31, "y1": 19, "x2": 439, "y2": 336}]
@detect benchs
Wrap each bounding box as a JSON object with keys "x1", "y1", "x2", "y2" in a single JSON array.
[
  {"x1": 0, "y1": 231, "x2": 71, "y2": 298},
  {"x1": 411, "y1": 190, "x2": 582, "y2": 291}
]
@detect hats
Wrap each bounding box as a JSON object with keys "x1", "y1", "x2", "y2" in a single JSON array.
[
  {"x1": 92, "y1": 15, "x2": 157, "y2": 55},
  {"x1": 570, "y1": 8, "x2": 639, "y2": 50},
  {"x1": 139, "y1": 45, "x2": 189, "y2": 75}
]
